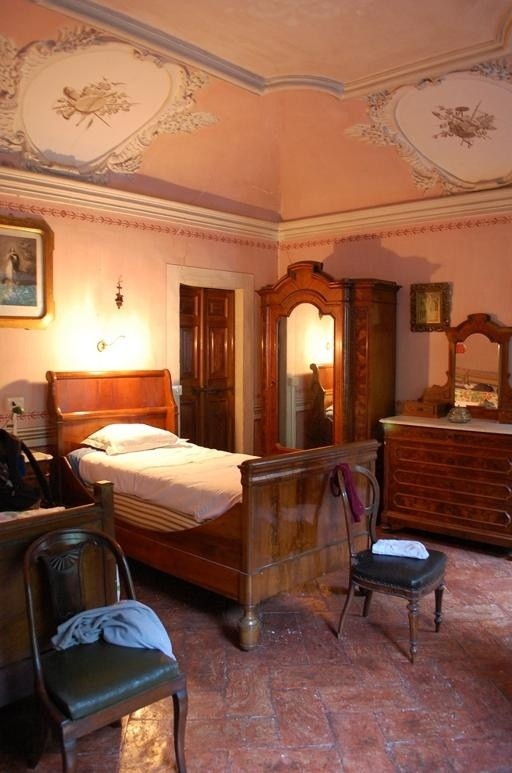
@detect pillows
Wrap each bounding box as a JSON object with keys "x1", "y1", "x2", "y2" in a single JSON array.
[{"x1": 81, "y1": 422, "x2": 190, "y2": 454}]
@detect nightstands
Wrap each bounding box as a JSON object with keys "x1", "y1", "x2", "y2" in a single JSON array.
[{"x1": 16, "y1": 447, "x2": 53, "y2": 491}]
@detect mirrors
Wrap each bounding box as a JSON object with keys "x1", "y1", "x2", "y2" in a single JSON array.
[
  {"x1": 276, "y1": 299, "x2": 335, "y2": 449},
  {"x1": 445, "y1": 309, "x2": 512, "y2": 423}
]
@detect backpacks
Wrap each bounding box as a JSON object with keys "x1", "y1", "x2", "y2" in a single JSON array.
[{"x1": 0, "y1": 430, "x2": 52, "y2": 512}]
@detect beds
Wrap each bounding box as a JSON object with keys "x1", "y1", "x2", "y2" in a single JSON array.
[{"x1": 43, "y1": 368, "x2": 378, "y2": 652}]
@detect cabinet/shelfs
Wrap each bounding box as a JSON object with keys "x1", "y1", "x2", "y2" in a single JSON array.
[
  {"x1": 252, "y1": 258, "x2": 399, "y2": 457},
  {"x1": 378, "y1": 413, "x2": 512, "y2": 560},
  {"x1": 0, "y1": 480, "x2": 120, "y2": 730}
]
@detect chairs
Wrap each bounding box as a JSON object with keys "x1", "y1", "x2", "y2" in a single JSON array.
[
  {"x1": 24, "y1": 526, "x2": 189, "y2": 773},
  {"x1": 329, "y1": 462, "x2": 448, "y2": 665}
]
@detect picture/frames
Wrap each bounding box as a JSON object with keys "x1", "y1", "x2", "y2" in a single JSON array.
[
  {"x1": 0, "y1": 215, "x2": 56, "y2": 330},
  {"x1": 408, "y1": 282, "x2": 447, "y2": 332}
]
